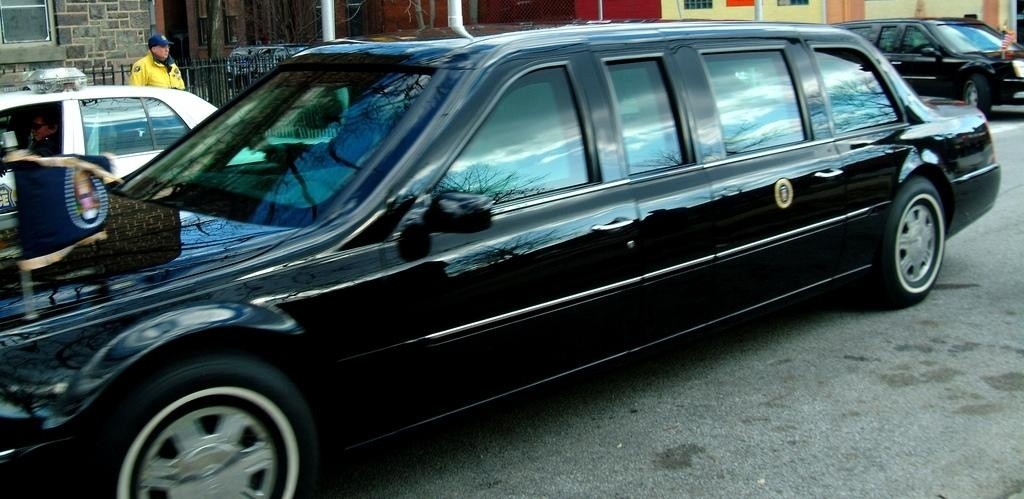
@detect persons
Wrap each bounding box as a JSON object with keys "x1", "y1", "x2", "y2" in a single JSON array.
[
  {"x1": 126, "y1": 32, "x2": 185, "y2": 90},
  {"x1": 25, "y1": 108, "x2": 61, "y2": 154}
]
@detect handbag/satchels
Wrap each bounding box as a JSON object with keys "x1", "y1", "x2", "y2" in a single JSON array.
[{"x1": 5, "y1": 147, "x2": 126, "y2": 273}]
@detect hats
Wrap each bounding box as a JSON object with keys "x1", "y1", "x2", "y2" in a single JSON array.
[{"x1": 148, "y1": 34, "x2": 175, "y2": 48}]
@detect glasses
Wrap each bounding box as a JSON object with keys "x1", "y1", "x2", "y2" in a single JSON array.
[{"x1": 32, "y1": 122, "x2": 45, "y2": 132}]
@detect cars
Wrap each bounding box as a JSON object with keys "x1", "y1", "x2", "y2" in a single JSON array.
[
  {"x1": 4, "y1": 21, "x2": 1004, "y2": 499},
  {"x1": 222, "y1": 42, "x2": 309, "y2": 86},
  {"x1": 1, "y1": 67, "x2": 267, "y2": 261},
  {"x1": 828, "y1": 17, "x2": 1024, "y2": 115}
]
